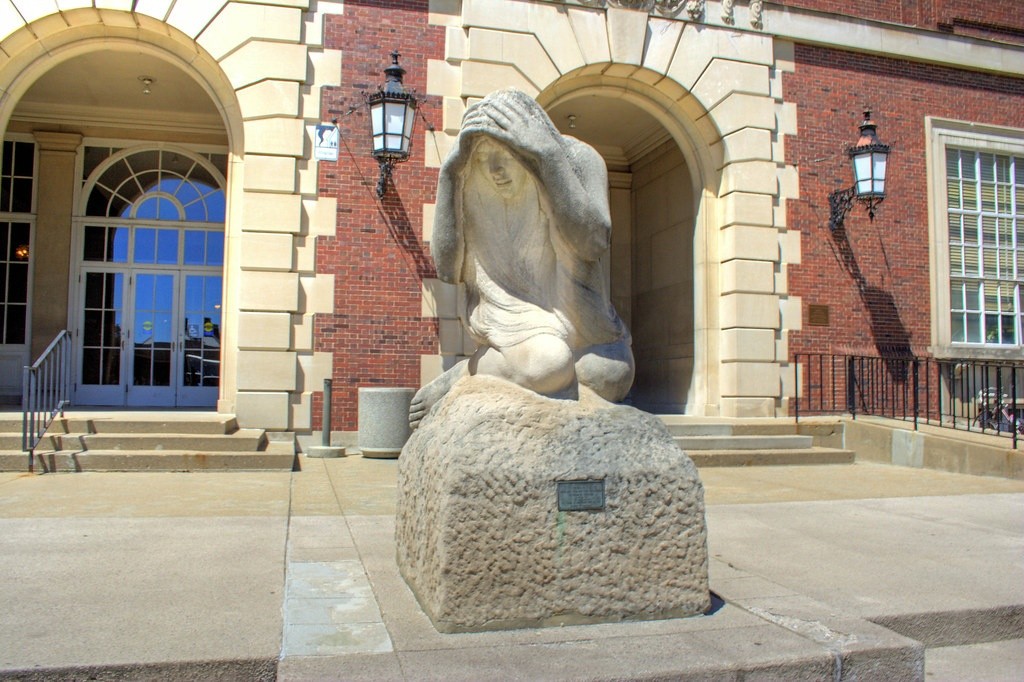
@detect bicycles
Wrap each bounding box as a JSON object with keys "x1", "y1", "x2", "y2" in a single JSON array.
[{"x1": 974, "y1": 385, "x2": 1024, "y2": 435}]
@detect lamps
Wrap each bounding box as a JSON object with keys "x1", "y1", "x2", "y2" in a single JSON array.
[
  {"x1": 829, "y1": 109, "x2": 891, "y2": 228},
  {"x1": 369, "y1": 50, "x2": 419, "y2": 198},
  {"x1": 568, "y1": 114, "x2": 576, "y2": 132},
  {"x1": 142, "y1": 79, "x2": 152, "y2": 95}
]
